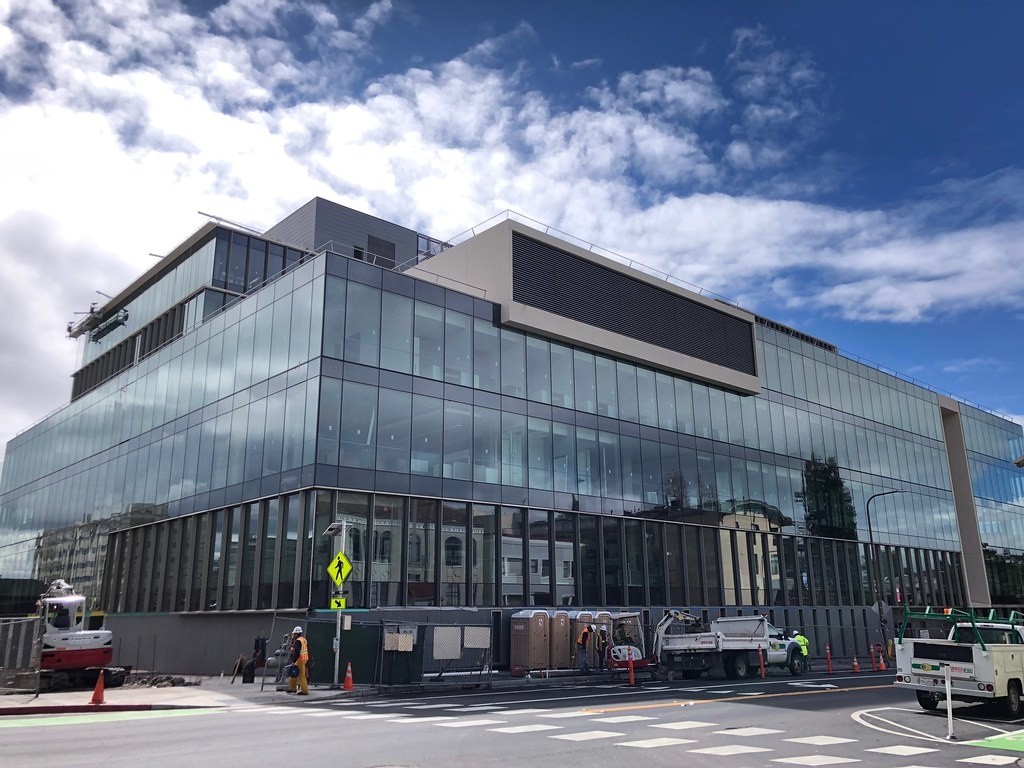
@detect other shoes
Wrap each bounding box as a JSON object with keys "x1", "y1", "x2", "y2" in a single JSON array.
[
  {"x1": 580, "y1": 669, "x2": 591, "y2": 671},
  {"x1": 297, "y1": 692, "x2": 308, "y2": 695},
  {"x1": 600, "y1": 669, "x2": 603, "y2": 672},
  {"x1": 607, "y1": 670, "x2": 612, "y2": 672},
  {"x1": 286, "y1": 690, "x2": 297, "y2": 693},
  {"x1": 801, "y1": 670, "x2": 807, "y2": 673}
]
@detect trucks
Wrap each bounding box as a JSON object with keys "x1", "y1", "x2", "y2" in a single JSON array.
[{"x1": 650, "y1": 609, "x2": 808, "y2": 681}]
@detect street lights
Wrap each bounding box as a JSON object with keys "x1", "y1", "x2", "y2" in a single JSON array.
[{"x1": 867, "y1": 488, "x2": 909, "y2": 667}]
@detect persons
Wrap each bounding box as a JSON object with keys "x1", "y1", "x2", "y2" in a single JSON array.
[
  {"x1": 793, "y1": 630, "x2": 810, "y2": 673},
  {"x1": 286, "y1": 626, "x2": 309, "y2": 695},
  {"x1": 577, "y1": 624, "x2": 597, "y2": 672},
  {"x1": 596, "y1": 625, "x2": 611, "y2": 669},
  {"x1": 615, "y1": 623, "x2": 635, "y2": 645}
]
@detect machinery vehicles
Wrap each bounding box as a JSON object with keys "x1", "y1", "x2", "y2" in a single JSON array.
[
  {"x1": 607, "y1": 607, "x2": 706, "y2": 675},
  {"x1": 31, "y1": 579, "x2": 129, "y2": 692}
]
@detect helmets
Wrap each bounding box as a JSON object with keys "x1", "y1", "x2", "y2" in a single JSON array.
[
  {"x1": 599, "y1": 625, "x2": 607, "y2": 631},
  {"x1": 291, "y1": 626, "x2": 303, "y2": 634},
  {"x1": 621, "y1": 620, "x2": 625, "y2": 623},
  {"x1": 591, "y1": 624, "x2": 596, "y2": 632},
  {"x1": 792, "y1": 630, "x2": 800, "y2": 637}
]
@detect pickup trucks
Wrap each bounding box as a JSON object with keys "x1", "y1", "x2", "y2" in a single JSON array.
[{"x1": 893, "y1": 603, "x2": 1024, "y2": 720}]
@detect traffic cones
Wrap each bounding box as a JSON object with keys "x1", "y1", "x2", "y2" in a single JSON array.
[
  {"x1": 878, "y1": 651, "x2": 887, "y2": 671},
  {"x1": 852, "y1": 655, "x2": 861, "y2": 673},
  {"x1": 89, "y1": 670, "x2": 109, "y2": 704},
  {"x1": 343, "y1": 661, "x2": 356, "y2": 691}
]
